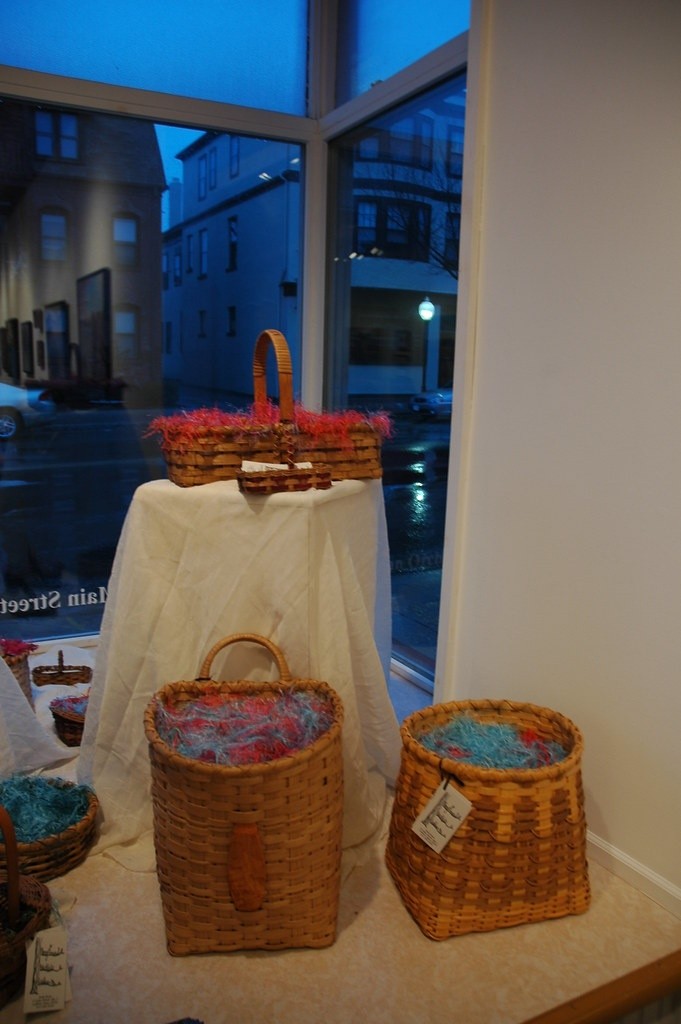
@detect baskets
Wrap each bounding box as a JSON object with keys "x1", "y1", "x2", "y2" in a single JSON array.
[
  {"x1": 162, "y1": 329, "x2": 384, "y2": 488},
  {"x1": 0, "y1": 639, "x2": 31, "y2": 704},
  {"x1": 49, "y1": 687, "x2": 90, "y2": 746},
  {"x1": 236, "y1": 424, "x2": 333, "y2": 494},
  {"x1": 0, "y1": 776, "x2": 99, "y2": 884},
  {"x1": 386, "y1": 700, "x2": 592, "y2": 940},
  {"x1": 145, "y1": 633, "x2": 344, "y2": 956},
  {"x1": 0, "y1": 806, "x2": 51, "y2": 1006},
  {"x1": 33, "y1": 650, "x2": 92, "y2": 687}
]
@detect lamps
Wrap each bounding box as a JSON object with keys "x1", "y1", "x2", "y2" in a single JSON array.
[{"x1": 417, "y1": 296, "x2": 435, "y2": 321}]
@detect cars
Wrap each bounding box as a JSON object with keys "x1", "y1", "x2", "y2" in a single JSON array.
[
  {"x1": 0, "y1": 383, "x2": 58, "y2": 444},
  {"x1": 409, "y1": 378, "x2": 454, "y2": 421}
]
